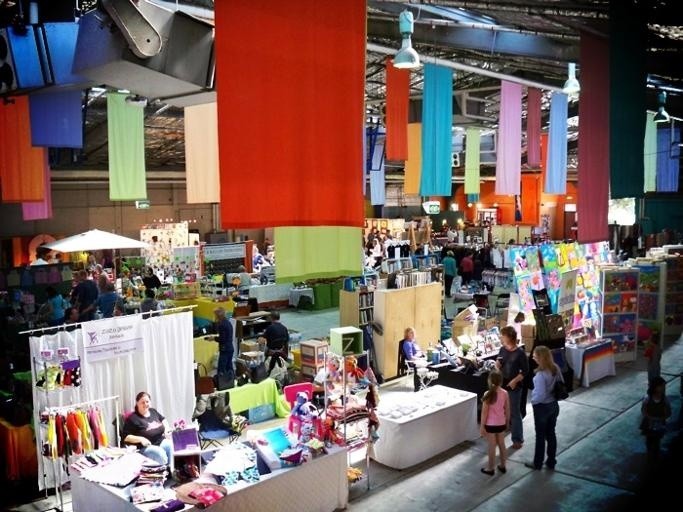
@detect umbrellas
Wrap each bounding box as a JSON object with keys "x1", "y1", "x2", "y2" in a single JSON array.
[{"x1": 40, "y1": 228, "x2": 150, "y2": 253}]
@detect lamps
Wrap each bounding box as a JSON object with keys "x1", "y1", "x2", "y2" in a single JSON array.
[
  {"x1": 390, "y1": 4, "x2": 423, "y2": 71},
  {"x1": 559, "y1": 62, "x2": 582, "y2": 96},
  {"x1": 650, "y1": 87, "x2": 670, "y2": 123}
]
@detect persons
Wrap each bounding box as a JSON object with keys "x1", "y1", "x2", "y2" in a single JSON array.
[
  {"x1": 27, "y1": 252, "x2": 163, "y2": 327},
  {"x1": 402, "y1": 327, "x2": 424, "y2": 366},
  {"x1": 366, "y1": 226, "x2": 393, "y2": 257},
  {"x1": 479, "y1": 369, "x2": 511, "y2": 476},
  {"x1": 495, "y1": 326, "x2": 530, "y2": 450},
  {"x1": 207, "y1": 306, "x2": 235, "y2": 375},
  {"x1": 264, "y1": 310, "x2": 290, "y2": 360},
  {"x1": 441, "y1": 250, "x2": 457, "y2": 298},
  {"x1": 238, "y1": 265, "x2": 261, "y2": 285},
  {"x1": 122, "y1": 391, "x2": 172, "y2": 466},
  {"x1": 459, "y1": 251, "x2": 474, "y2": 286},
  {"x1": 447, "y1": 227, "x2": 457, "y2": 243},
  {"x1": 523, "y1": 345, "x2": 564, "y2": 470},
  {"x1": 252, "y1": 246, "x2": 264, "y2": 270}
]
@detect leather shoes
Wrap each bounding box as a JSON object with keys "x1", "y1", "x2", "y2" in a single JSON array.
[
  {"x1": 497, "y1": 465, "x2": 506, "y2": 473},
  {"x1": 481, "y1": 468, "x2": 495, "y2": 476},
  {"x1": 524, "y1": 462, "x2": 541, "y2": 470}
]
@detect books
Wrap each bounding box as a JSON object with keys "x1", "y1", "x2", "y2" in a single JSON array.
[
  {"x1": 359, "y1": 293, "x2": 373, "y2": 324},
  {"x1": 387, "y1": 267, "x2": 431, "y2": 289}
]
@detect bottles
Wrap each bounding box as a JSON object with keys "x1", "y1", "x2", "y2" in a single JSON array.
[
  {"x1": 426, "y1": 339, "x2": 443, "y2": 362},
  {"x1": 481, "y1": 269, "x2": 509, "y2": 289}
]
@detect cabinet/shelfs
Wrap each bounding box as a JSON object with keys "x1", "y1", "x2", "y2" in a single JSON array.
[
  {"x1": 317, "y1": 347, "x2": 373, "y2": 494},
  {"x1": 338, "y1": 245, "x2": 682, "y2": 415}
]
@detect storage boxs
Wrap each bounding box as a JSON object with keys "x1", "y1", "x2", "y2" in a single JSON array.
[{"x1": 237, "y1": 339, "x2": 328, "y2": 385}]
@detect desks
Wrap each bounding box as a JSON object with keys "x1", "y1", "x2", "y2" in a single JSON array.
[
  {"x1": 67, "y1": 427, "x2": 353, "y2": 511},
  {"x1": 363, "y1": 383, "x2": 478, "y2": 470},
  {"x1": 165, "y1": 277, "x2": 303, "y2": 324}
]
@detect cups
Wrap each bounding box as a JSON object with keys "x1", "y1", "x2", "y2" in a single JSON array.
[{"x1": 431, "y1": 351, "x2": 440, "y2": 364}]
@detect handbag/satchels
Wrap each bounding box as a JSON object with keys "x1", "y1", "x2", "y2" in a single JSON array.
[{"x1": 553, "y1": 381, "x2": 569, "y2": 400}]
